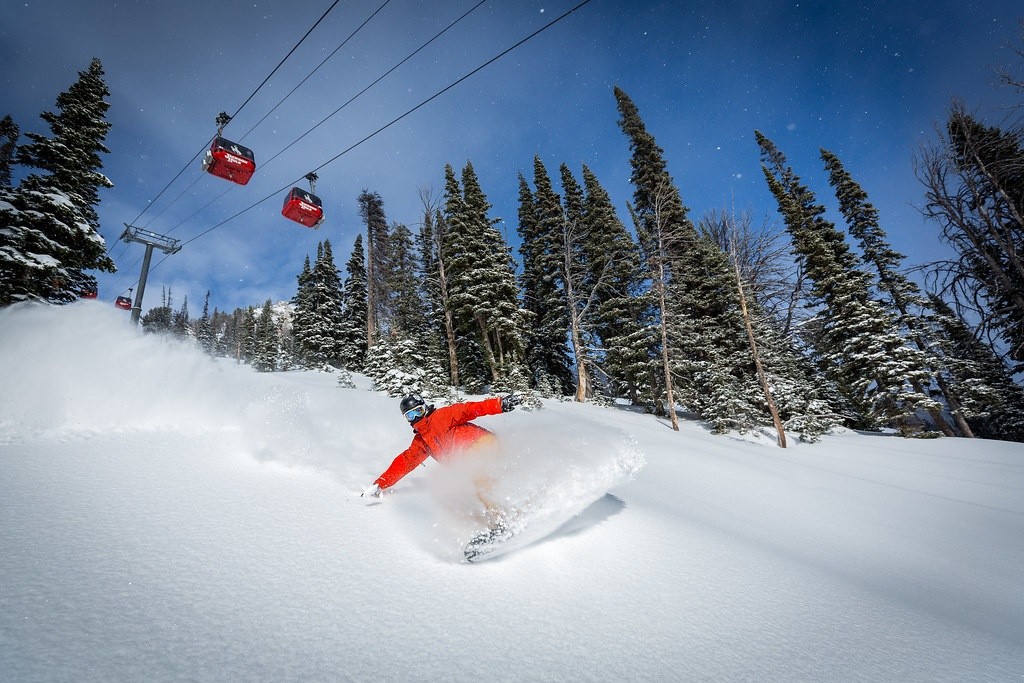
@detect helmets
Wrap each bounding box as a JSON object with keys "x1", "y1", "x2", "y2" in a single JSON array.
[{"x1": 400, "y1": 396, "x2": 424, "y2": 413}]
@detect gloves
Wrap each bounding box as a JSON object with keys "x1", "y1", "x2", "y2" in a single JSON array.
[{"x1": 501, "y1": 394, "x2": 522, "y2": 412}]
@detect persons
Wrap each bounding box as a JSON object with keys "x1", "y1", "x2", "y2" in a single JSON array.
[{"x1": 359, "y1": 391, "x2": 525, "y2": 536}]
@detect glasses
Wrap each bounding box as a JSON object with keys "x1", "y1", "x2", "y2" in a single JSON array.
[{"x1": 403, "y1": 404, "x2": 426, "y2": 421}]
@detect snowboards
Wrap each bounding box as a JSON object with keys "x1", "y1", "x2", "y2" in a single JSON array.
[{"x1": 463, "y1": 517, "x2": 542, "y2": 565}]
